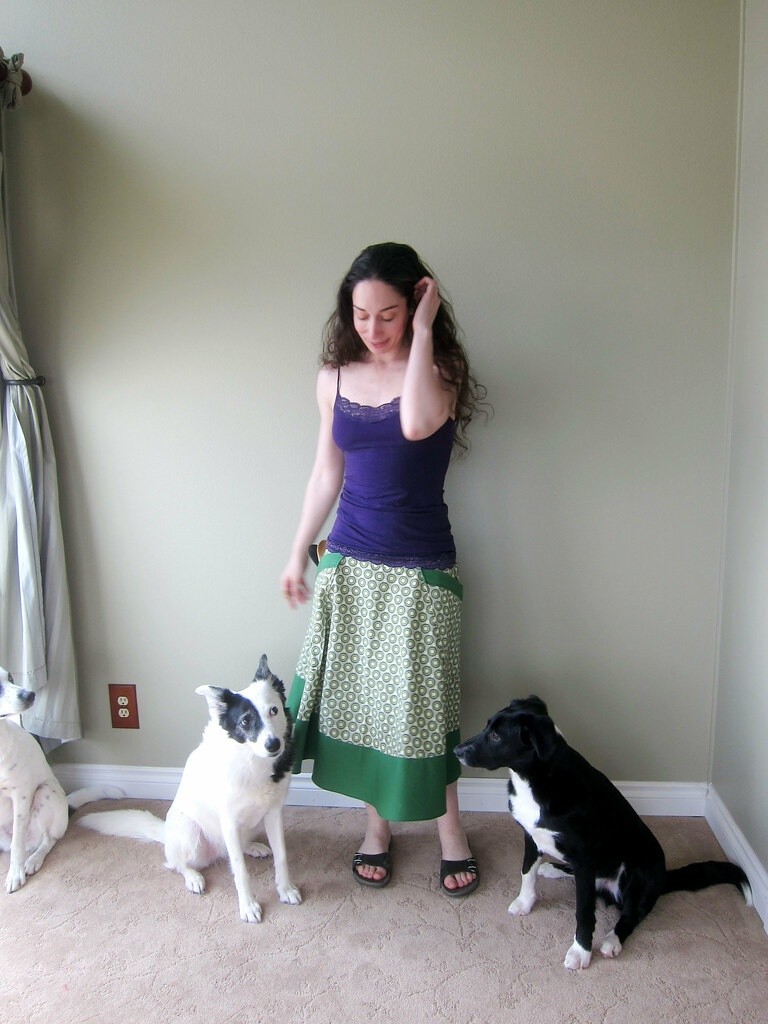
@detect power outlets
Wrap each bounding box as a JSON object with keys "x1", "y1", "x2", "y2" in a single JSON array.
[{"x1": 107, "y1": 683, "x2": 139, "y2": 730}]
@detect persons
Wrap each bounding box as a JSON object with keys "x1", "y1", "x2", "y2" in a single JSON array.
[{"x1": 280, "y1": 242, "x2": 493, "y2": 901}]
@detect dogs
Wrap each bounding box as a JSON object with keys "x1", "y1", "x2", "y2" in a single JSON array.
[
  {"x1": 0, "y1": 663, "x2": 125, "y2": 896},
  {"x1": 452, "y1": 695, "x2": 753, "y2": 971},
  {"x1": 73, "y1": 654, "x2": 304, "y2": 925}
]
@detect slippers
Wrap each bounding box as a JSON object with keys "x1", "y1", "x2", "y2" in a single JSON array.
[
  {"x1": 440, "y1": 837, "x2": 480, "y2": 897},
  {"x1": 352, "y1": 833, "x2": 394, "y2": 887}
]
